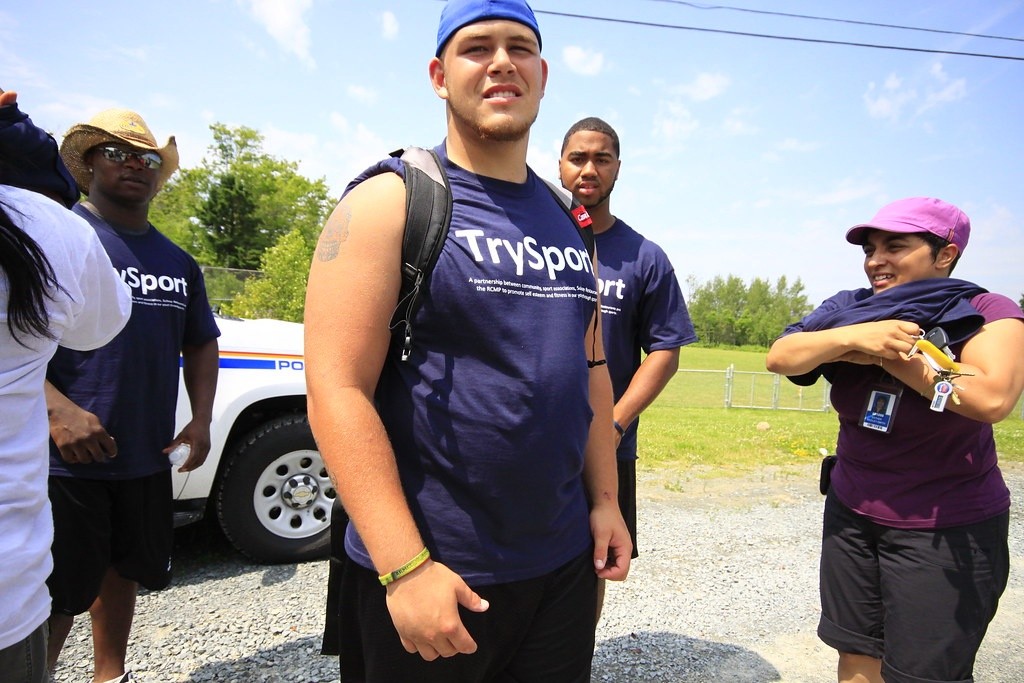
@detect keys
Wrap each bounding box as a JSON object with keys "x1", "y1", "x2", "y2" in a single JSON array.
[{"x1": 909, "y1": 326, "x2": 975, "y2": 412}]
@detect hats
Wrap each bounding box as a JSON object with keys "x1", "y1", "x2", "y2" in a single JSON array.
[
  {"x1": 435, "y1": 0, "x2": 543, "y2": 59},
  {"x1": 845, "y1": 197, "x2": 970, "y2": 256},
  {"x1": 57, "y1": 109, "x2": 178, "y2": 202}
]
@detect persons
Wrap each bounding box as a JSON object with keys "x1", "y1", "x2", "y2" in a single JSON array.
[
  {"x1": 44, "y1": 107, "x2": 222, "y2": 683},
  {"x1": 0, "y1": 86, "x2": 137, "y2": 683},
  {"x1": 559, "y1": 116, "x2": 698, "y2": 624},
  {"x1": 302, "y1": 0, "x2": 634, "y2": 683},
  {"x1": 765, "y1": 196, "x2": 1024, "y2": 682}
]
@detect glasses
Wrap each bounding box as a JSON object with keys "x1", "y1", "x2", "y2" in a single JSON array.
[{"x1": 85, "y1": 144, "x2": 163, "y2": 170}]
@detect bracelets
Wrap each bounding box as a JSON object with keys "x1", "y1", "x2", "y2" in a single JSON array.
[
  {"x1": 378, "y1": 543, "x2": 430, "y2": 586},
  {"x1": 880, "y1": 356, "x2": 883, "y2": 366},
  {"x1": 612, "y1": 418, "x2": 627, "y2": 438}
]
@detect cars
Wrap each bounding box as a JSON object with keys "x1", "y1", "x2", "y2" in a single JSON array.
[{"x1": 160, "y1": 310, "x2": 344, "y2": 564}]
